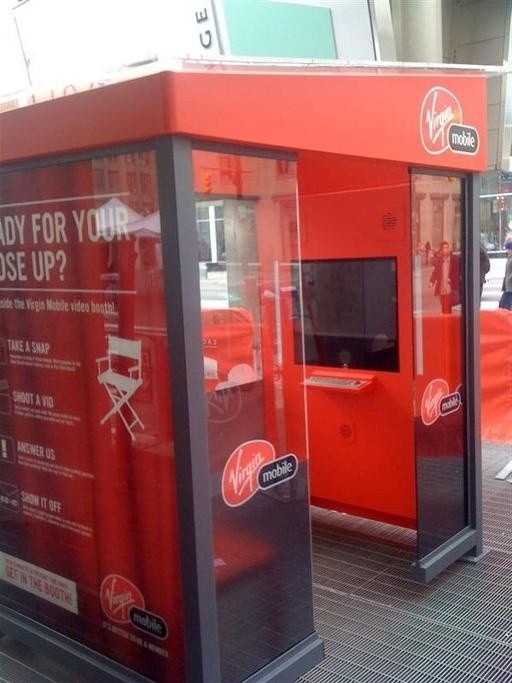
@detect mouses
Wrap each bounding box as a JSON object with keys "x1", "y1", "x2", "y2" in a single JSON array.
[{"x1": 353, "y1": 381, "x2": 361, "y2": 385}]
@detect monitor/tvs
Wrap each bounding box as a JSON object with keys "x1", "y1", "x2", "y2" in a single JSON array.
[{"x1": 290, "y1": 255, "x2": 399, "y2": 373}]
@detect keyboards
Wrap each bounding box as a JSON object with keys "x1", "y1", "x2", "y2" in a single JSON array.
[{"x1": 309, "y1": 377, "x2": 353, "y2": 386}]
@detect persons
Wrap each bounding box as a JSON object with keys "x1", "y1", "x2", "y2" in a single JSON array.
[
  {"x1": 480, "y1": 245, "x2": 490, "y2": 300},
  {"x1": 498, "y1": 241, "x2": 512, "y2": 311},
  {"x1": 429, "y1": 243, "x2": 461, "y2": 314}
]
